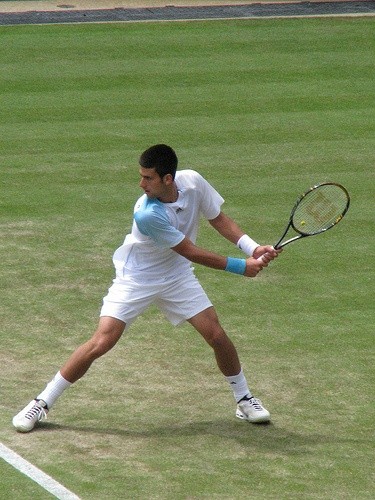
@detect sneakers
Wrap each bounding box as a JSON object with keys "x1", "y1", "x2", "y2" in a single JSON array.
[
  {"x1": 13, "y1": 396, "x2": 49, "y2": 432},
  {"x1": 235, "y1": 393, "x2": 270, "y2": 423}
]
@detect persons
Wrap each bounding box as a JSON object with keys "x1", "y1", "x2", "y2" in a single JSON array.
[{"x1": 12, "y1": 144, "x2": 283, "y2": 432}]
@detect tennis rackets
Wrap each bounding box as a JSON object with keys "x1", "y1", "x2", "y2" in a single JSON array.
[{"x1": 257, "y1": 182, "x2": 351, "y2": 263}]
